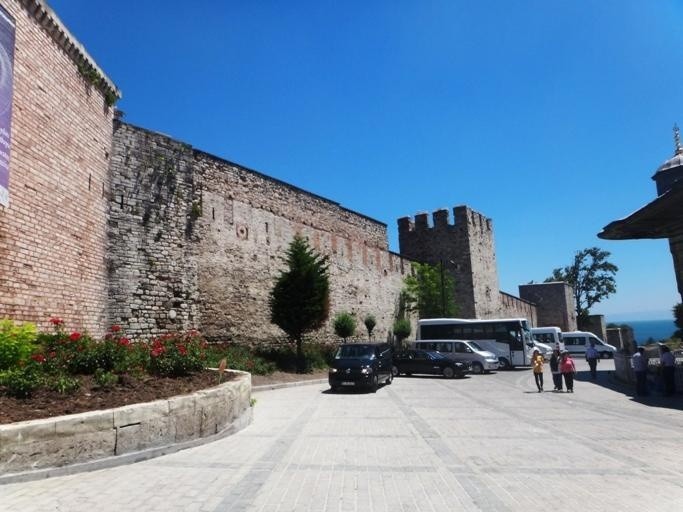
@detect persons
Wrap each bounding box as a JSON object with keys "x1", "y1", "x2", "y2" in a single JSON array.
[
  {"x1": 530, "y1": 349, "x2": 577, "y2": 393},
  {"x1": 632, "y1": 344, "x2": 675, "y2": 398},
  {"x1": 585, "y1": 338, "x2": 600, "y2": 376}
]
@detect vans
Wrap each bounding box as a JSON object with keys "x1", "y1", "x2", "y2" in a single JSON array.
[
  {"x1": 327, "y1": 342, "x2": 393, "y2": 394},
  {"x1": 530, "y1": 327, "x2": 617, "y2": 362},
  {"x1": 410, "y1": 339, "x2": 500, "y2": 375}
]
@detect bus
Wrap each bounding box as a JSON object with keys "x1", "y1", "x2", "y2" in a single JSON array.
[{"x1": 416, "y1": 317, "x2": 535, "y2": 371}]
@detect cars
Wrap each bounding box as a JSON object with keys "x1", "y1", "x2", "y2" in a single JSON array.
[{"x1": 390, "y1": 348, "x2": 473, "y2": 379}]
